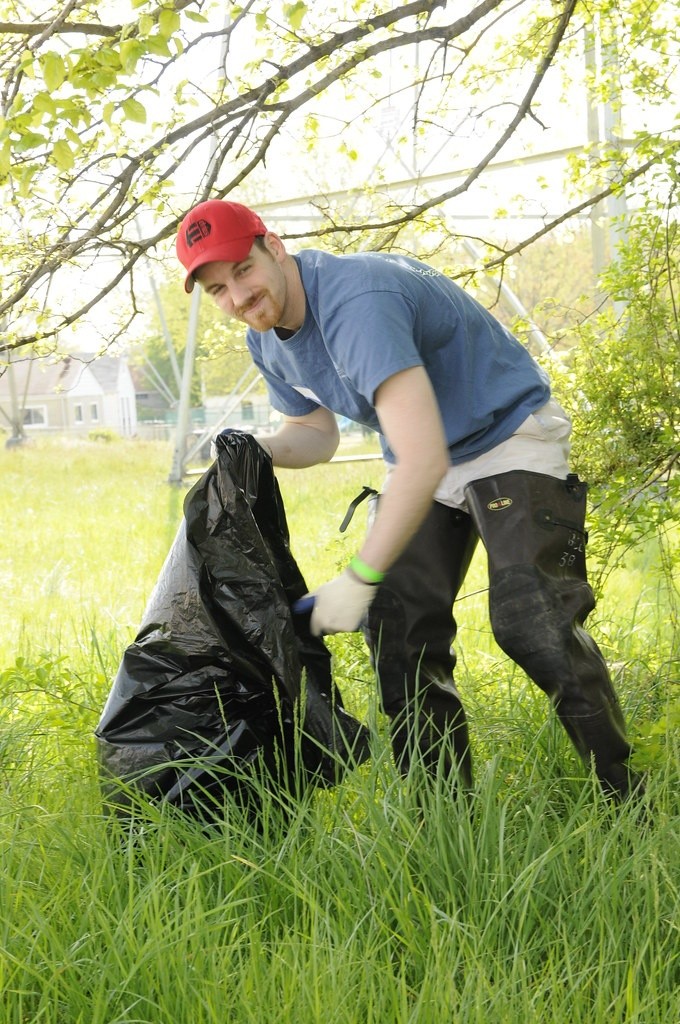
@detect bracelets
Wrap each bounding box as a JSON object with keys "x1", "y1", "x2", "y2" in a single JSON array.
[
  {"x1": 262, "y1": 438, "x2": 274, "y2": 458},
  {"x1": 347, "y1": 557, "x2": 383, "y2": 588}
]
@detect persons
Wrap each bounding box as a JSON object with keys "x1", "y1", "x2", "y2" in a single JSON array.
[{"x1": 176, "y1": 201, "x2": 646, "y2": 815}]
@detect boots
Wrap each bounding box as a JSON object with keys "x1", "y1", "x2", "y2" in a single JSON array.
[
  {"x1": 462, "y1": 470, "x2": 658, "y2": 855},
  {"x1": 339, "y1": 485, "x2": 479, "y2": 830}
]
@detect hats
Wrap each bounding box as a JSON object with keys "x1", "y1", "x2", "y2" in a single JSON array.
[{"x1": 175, "y1": 199, "x2": 269, "y2": 294}]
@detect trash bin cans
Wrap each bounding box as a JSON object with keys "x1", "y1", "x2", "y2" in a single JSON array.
[{"x1": 193, "y1": 429, "x2": 211, "y2": 460}]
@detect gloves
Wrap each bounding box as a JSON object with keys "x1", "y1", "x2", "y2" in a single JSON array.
[
  {"x1": 210, "y1": 427, "x2": 273, "y2": 462},
  {"x1": 301, "y1": 569, "x2": 379, "y2": 637}
]
